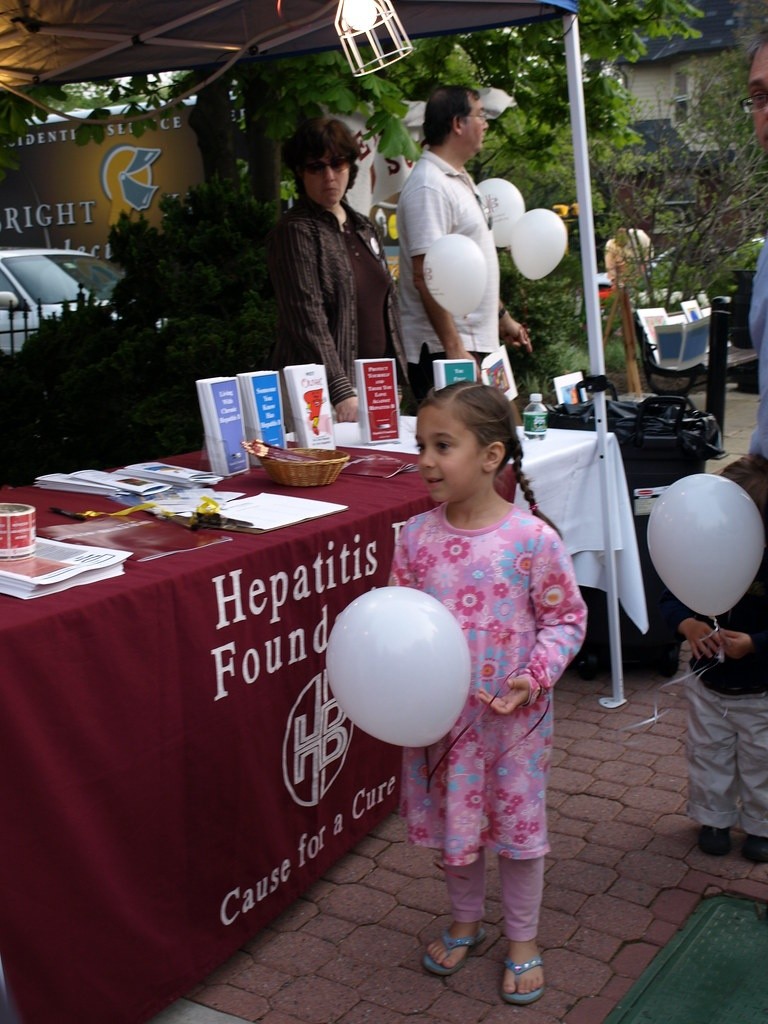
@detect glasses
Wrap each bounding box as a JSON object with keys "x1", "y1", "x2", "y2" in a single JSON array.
[
  {"x1": 740, "y1": 93, "x2": 768, "y2": 114},
  {"x1": 305, "y1": 155, "x2": 350, "y2": 175},
  {"x1": 461, "y1": 113, "x2": 486, "y2": 122}
]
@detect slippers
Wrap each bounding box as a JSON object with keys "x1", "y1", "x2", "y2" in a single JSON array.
[
  {"x1": 500, "y1": 955, "x2": 544, "y2": 1004},
  {"x1": 423, "y1": 927, "x2": 486, "y2": 974}
]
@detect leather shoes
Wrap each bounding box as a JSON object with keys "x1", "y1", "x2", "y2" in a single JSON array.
[
  {"x1": 699, "y1": 826, "x2": 730, "y2": 856},
  {"x1": 741, "y1": 835, "x2": 768, "y2": 862}
]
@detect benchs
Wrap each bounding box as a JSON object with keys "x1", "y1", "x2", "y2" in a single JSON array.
[{"x1": 635, "y1": 304, "x2": 757, "y2": 396}]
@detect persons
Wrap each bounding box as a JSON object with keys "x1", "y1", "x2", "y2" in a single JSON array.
[
  {"x1": 740, "y1": 28, "x2": 768, "y2": 457},
  {"x1": 398, "y1": 83, "x2": 533, "y2": 405},
  {"x1": 603, "y1": 228, "x2": 655, "y2": 288},
  {"x1": 660, "y1": 454, "x2": 768, "y2": 862},
  {"x1": 264, "y1": 116, "x2": 410, "y2": 423},
  {"x1": 384, "y1": 381, "x2": 588, "y2": 1005}
]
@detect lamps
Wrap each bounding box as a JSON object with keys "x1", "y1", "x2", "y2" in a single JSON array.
[{"x1": 334, "y1": 0, "x2": 415, "y2": 77}]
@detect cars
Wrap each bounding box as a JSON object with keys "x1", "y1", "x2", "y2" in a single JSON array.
[{"x1": 0, "y1": 248, "x2": 171, "y2": 354}]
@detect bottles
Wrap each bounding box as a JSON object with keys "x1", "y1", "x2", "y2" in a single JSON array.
[{"x1": 522, "y1": 393, "x2": 548, "y2": 440}]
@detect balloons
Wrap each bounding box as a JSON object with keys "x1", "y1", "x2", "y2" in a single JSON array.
[
  {"x1": 326, "y1": 586, "x2": 470, "y2": 749},
  {"x1": 423, "y1": 234, "x2": 486, "y2": 316},
  {"x1": 647, "y1": 472, "x2": 765, "y2": 627},
  {"x1": 474, "y1": 178, "x2": 568, "y2": 280}
]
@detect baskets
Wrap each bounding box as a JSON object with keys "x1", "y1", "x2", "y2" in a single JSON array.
[{"x1": 257, "y1": 447, "x2": 352, "y2": 487}]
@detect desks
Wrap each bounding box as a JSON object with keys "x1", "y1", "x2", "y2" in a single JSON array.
[{"x1": 0, "y1": 415, "x2": 617, "y2": 1024}]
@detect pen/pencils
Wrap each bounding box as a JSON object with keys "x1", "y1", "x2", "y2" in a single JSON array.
[{"x1": 48, "y1": 506, "x2": 86, "y2": 521}]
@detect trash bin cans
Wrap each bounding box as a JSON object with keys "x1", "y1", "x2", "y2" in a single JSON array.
[{"x1": 546, "y1": 378, "x2": 718, "y2": 682}]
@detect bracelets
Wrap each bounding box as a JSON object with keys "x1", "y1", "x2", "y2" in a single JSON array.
[{"x1": 499, "y1": 303, "x2": 508, "y2": 318}]
